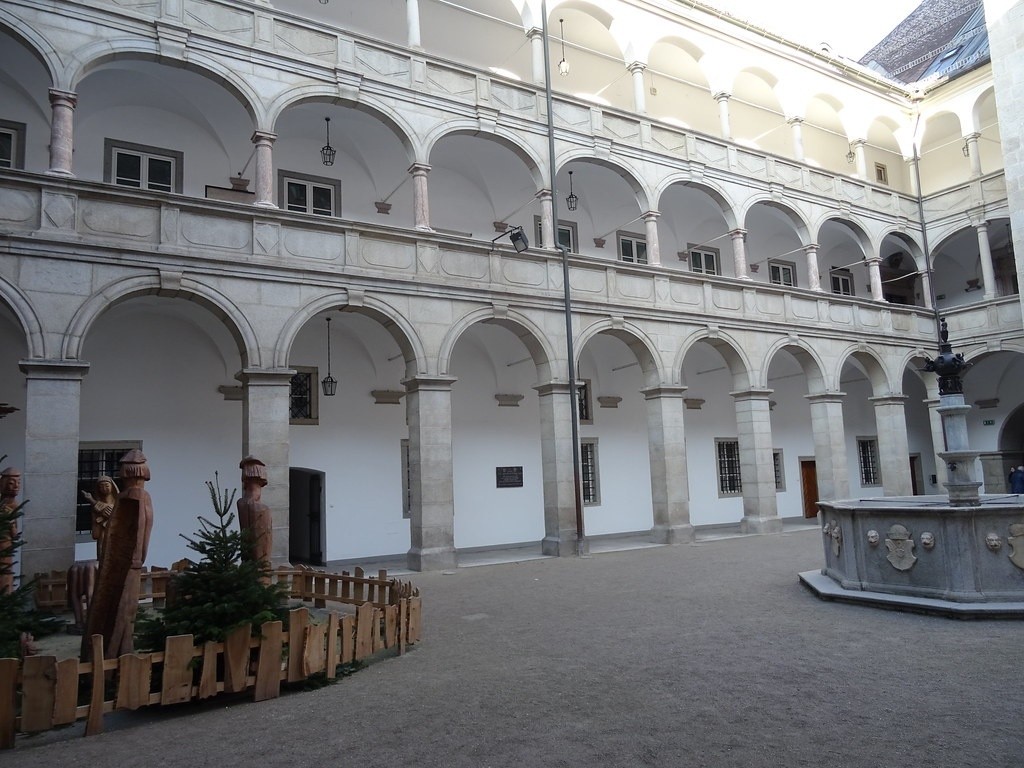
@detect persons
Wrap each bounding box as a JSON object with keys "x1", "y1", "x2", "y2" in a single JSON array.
[
  {"x1": 1008, "y1": 466, "x2": 1024, "y2": 494},
  {"x1": 237, "y1": 457, "x2": 274, "y2": 589},
  {"x1": 0, "y1": 466, "x2": 21, "y2": 594},
  {"x1": 82, "y1": 474, "x2": 120, "y2": 560},
  {"x1": 82, "y1": 449, "x2": 153, "y2": 661}
]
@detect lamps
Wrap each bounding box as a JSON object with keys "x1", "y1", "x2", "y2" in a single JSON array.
[
  {"x1": 862, "y1": 256, "x2": 869, "y2": 267},
  {"x1": 577, "y1": 359, "x2": 587, "y2": 402},
  {"x1": 491, "y1": 225, "x2": 529, "y2": 254},
  {"x1": 1005, "y1": 223, "x2": 1014, "y2": 255},
  {"x1": 846, "y1": 142, "x2": 856, "y2": 165},
  {"x1": 320, "y1": 117, "x2": 336, "y2": 166},
  {"x1": 558, "y1": 19, "x2": 570, "y2": 77},
  {"x1": 566, "y1": 170, "x2": 578, "y2": 212},
  {"x1": 962, "y1": 140, "x2": 971, "y2": 157},
  {"x1": 321, "y1": 317, "x2": 337, "y2": 396}
]
